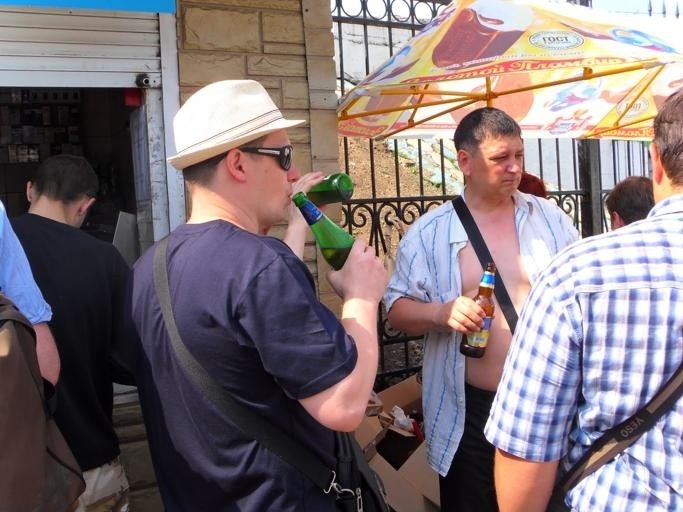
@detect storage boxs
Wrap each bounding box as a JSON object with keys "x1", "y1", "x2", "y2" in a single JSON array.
[{"x1": 347, "y1": 372, "x2": 449, "y2": 512}]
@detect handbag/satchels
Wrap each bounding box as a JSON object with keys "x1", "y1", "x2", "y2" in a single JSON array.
[{"x1": 337, "y1": 431, "x2": 390, "y2": 512}]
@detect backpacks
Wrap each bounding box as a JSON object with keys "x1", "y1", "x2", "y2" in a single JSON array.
[{"x1": 0, "y1": 293, "x2": 86, "y2": 512}]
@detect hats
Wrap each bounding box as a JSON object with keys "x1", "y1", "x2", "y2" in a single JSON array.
[{"x1": 166, "y1": 79, "x2": 306, "y2": 170}]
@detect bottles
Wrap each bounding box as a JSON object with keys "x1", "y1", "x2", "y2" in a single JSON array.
[
  {"x1": 459, "y1": 263, "x2": 497, "y2": 358},
  {"x1": 291, "y1": 191, "x2": 355, "y2": 271},
  {"x1": 305, "y1": 173, "x2": 353, "y2": 207}
]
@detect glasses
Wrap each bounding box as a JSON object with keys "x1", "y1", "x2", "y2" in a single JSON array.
[{"x1": 240, "y1": 145, "x2": 293, "y2": 170}]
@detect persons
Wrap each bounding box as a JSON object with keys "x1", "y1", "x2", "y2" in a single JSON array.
[
  {"x1": 605, "y1": 175, "x2": 655, "y2": 231},
  {"x1": 7, "y1": 152, "x2": 138, "y2": 512},
  {"x1": 280, "y1": 171, "x2": 323, "y2": 264},
  {"x1": 482, "y1": 84, "x2": 680, "y2": 512},
  {"x1": 382, "y1": 106, "x2": 583, "y2": 512},
  {"x1": 108, "y1": 79, "x2": 393, "y2": 511},
  {"x1": 0, "y1": 198, "x2": 59, "y2": 400}
]
